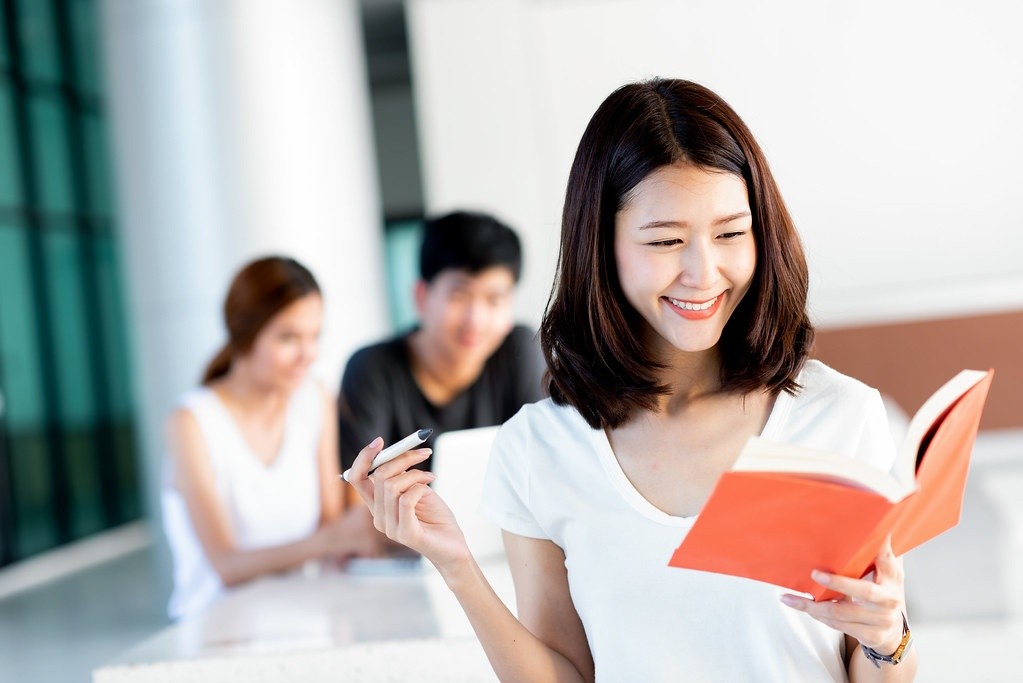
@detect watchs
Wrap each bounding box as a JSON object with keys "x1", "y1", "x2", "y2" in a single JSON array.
[{"x1": 861, "y1": 611, "x2": 916, "y2": 667}]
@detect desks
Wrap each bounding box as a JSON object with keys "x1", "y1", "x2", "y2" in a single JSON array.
[{"x1": 97, "y1": 568, "x2": 519, "y2": 682}]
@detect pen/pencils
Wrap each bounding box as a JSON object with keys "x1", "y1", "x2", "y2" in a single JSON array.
[{"x1": 341, "y1": 428, "x2": 433, "y2": 484}]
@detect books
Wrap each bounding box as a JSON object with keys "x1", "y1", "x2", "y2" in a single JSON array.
[{"x1": 665, "y1": 367, "x2": 996, "y2": 602}]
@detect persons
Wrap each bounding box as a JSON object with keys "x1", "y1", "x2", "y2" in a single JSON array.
[
  {"x1": 156, "y1": 255, "x2": 390, "y2": 621},
  {"x1": 349, "y1": 74, "x2": 915, "y2": 683},
  {"x1": 335, "y1": 211, "x2": 545, "y2": 473}
]
